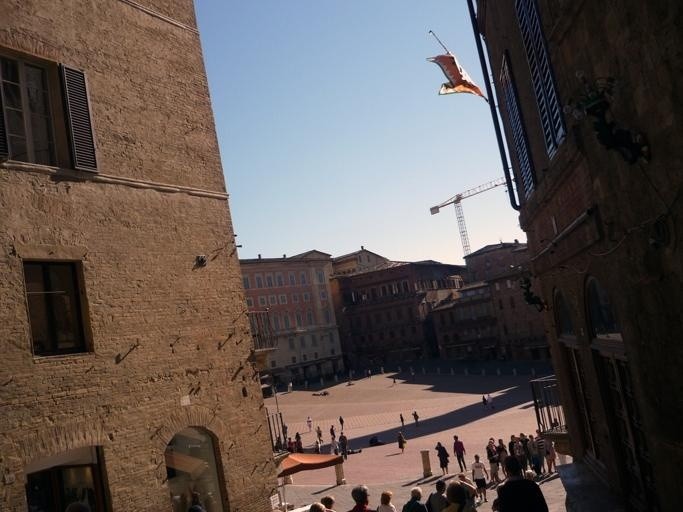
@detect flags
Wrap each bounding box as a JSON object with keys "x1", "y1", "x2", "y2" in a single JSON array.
[{"x1": 427, "y1": 49, "x2": 483, "y2": 98}]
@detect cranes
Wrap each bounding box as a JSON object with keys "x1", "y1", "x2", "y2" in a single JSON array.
[{"x1": 429, "y1": 172, "x2": 522, "y2": 257}]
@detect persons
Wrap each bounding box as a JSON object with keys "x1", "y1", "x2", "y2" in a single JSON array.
[
  {"x1": 287, "y1": 415, "x2": 567, "y2": 511},
  {"x1": 482, "y1": 395, "x2": 487, "y2": 409},
  {"x1": 367, "y1": 368, "x2": 371, "y2": 380},
  {"x1": 392, "y1": 376, "x2": 399, "y2": 392},
  {"x1": 170, "y1": 488, "x2": 218, "y2": 512},
  {"x1": 412, "y1": 410, "x2": 419, "y2": 427},
  {"x1": 486, "y1": 394, "x2": 493, "y2": 410},
  {"x1": 399, "y1": 413, "x2": 404, "y2": 427},
  {"x1": 411, "y1": 367, "x2": 416, "y2": 381}
]
